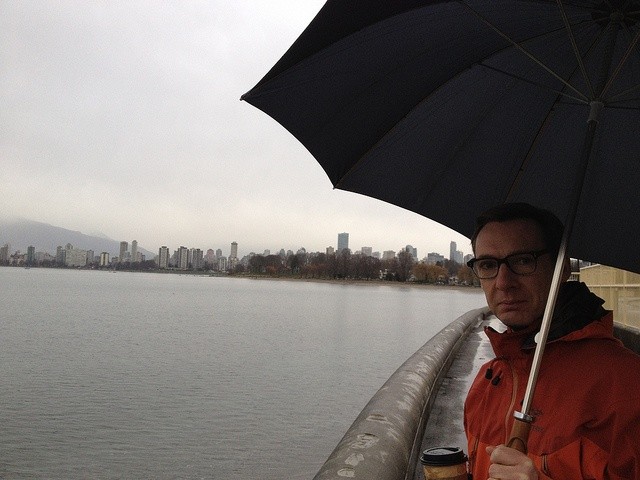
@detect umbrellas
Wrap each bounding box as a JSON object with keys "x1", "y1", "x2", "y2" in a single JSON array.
[{"x1": 241, "y1": 0, "x2": 640, "y2": 453}]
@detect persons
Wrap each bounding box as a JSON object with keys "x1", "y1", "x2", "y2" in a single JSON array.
[{"x1": 465, "y1": 203, "x2": 640, "y2": 480}]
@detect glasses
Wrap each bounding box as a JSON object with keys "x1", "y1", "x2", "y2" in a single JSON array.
[{"x1": 468, "y1": 251, "x2": 553, "y2": 279}]
